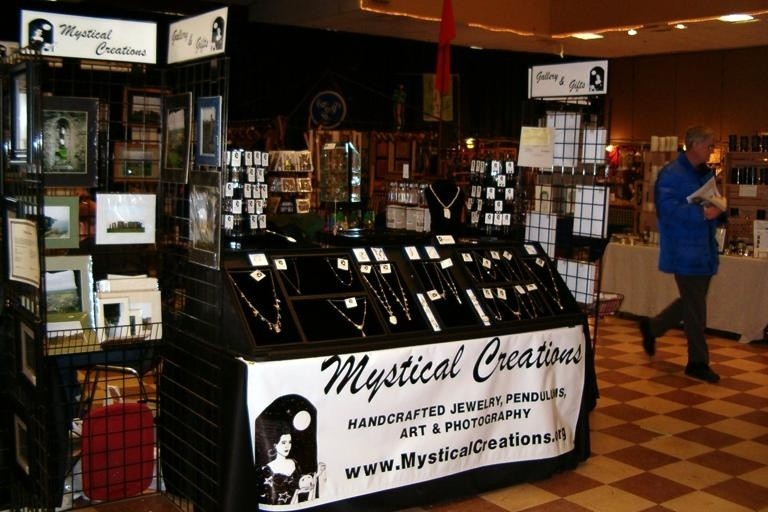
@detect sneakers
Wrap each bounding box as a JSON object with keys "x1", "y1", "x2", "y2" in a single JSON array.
[
  {"x1": 640, "y1": 316, "x2": 655, "y2": 357},
  {"x1": 685, "y1": 361, "x2": 720, "y2": 384}
]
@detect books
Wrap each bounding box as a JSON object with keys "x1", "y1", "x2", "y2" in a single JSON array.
[
  {"x1": 52, "y1": 200, "x2": 162, "y2": 440},
  {"x1": 686, "y1": 175, "x2": 728, "y2": 212}
]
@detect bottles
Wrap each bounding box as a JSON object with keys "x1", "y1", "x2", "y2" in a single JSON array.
[
  {"x1": 416, "y1": 184, "x2": 431, "y2": 232},
  {"x1": 396, "y1": 184, "x2": 405, "y2": 229},
  {"x1": 414, "y1": 184, "x2": 419, "y2": 192},
  {"x1": 405, "y1": 184, "x2": 416, "y2": 230},
  {"x1": 405, "y1": 183, "x2": 409, "y2": 193},
  {"x1": 386, "y1": 182, "x2": 397, "y2": 228}
]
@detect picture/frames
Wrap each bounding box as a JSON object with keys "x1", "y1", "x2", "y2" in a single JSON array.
[
  {"x1": 95, "y1": 298, "x2": 131, "y2": 341},
  {"x1": 39, "y1": 96, "x2": 98, "y2": 188},
  {"x1": 128, "y1": 308, "x2": 142, "y2": 338},
  {"x1": 187, "y1": 171, "x2": 222, "y2": 271},
  {"x1": 4, "y1": 61, "x2": 33, "y2": 165},
  {"x1": 40, "y1": 254, "x2": 96, "y2": 331},
  {"x1": 161, "y1": 91, "x2": 193, "y2": 185},
  {"x1": 21, "y1": 321, "x2": 36, "y2": 387},
  {"x1": 97, "y1": 292, "x2": 163, "y2": 340},
  {"x1": 195, "y1": 95, "x2": 222, "y2": 167},
  {"x1": 43, "y1": 312, "x2": 100, "y2": 355},
  {"x1": 16, "y1": 196, "x2": 80, "y2": 249},
  {"x1": 14, "y1": 413, "x2": 31, "y2": 476}
]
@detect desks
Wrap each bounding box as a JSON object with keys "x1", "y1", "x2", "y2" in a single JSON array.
[{"x1": 595, "y1": 232, "x2": 768, "y2": 344}]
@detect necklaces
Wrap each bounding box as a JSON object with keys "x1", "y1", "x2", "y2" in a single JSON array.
[
  {"x1": 359, "y1": 247, "x2": 564, "y2": 326},
  {"x1": 429, "y1": 184, "x2": 460, "y2": 220},
  {"x1": 324, "y1": 256, "x2": 353, "y2": 286},
  {"x1": 226, "y1": 267, "x2": 282, "y2": 334},
  {"x1": 326, "y1": 296, "x2": 367, "y2": 337},
  {"x1": 281, "y1": 259, "x2": 302, "y2": 296}
]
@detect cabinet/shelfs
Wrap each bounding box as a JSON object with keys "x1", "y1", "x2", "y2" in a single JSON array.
[
  {"x1": 725, "y1": 152, "x2": 768, "y2": 245},
  {"x1": 156, "y1": 237, "x2": 601, "y2": 511},
  {"x1": 521, "y1": 95, "x2": 624, "y2": 361},
  {"x1": 0, "y1": 40, "x2": 232, "y2": 512}
]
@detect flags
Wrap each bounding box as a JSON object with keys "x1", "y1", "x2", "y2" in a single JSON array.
[{"x1": 434, "y1": 0, "x2": 456, "y2": 95}]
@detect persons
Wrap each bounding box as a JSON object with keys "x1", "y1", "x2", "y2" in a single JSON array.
[
  {"x1": 623, "y1": 161, "x2": 642, "y2": 201},
  {"x1": 263, "y1": 427, "x2": 326, "y2": 505},
  {"x1": 639, "y1": 126, "x2": 727, "y2": 383}
]
